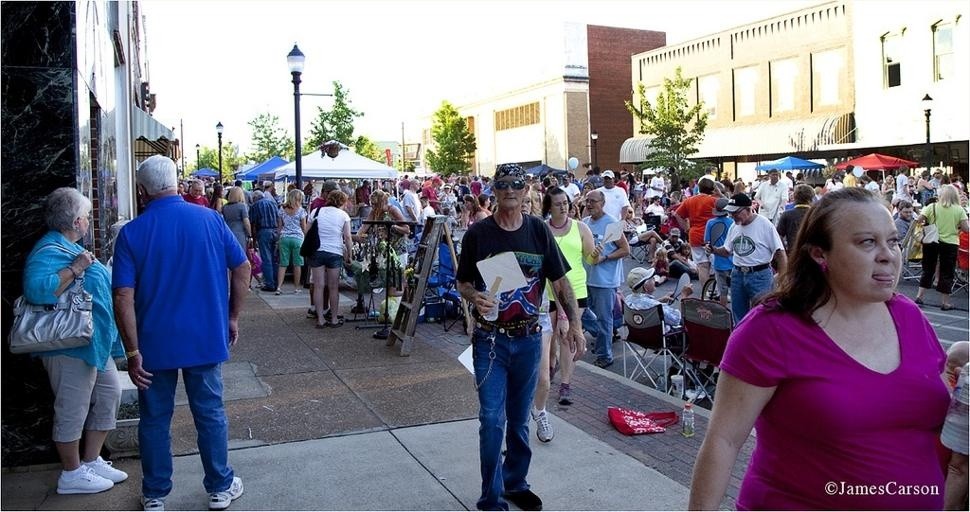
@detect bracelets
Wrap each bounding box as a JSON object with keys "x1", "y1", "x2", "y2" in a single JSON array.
[
  {"x1": 125, "y1": 349, "x2": 140, "y2": 360},
  {"x1": 67, "y1": 265, "x2": 77, "y2": 278}
]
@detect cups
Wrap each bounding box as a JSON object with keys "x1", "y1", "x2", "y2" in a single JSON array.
[
  {"x1": 671, "y1": 375, "x2": 684, "y2": 399},
  {"x1": 483, "y1": 290, "x2": 500, "y2": 321}
]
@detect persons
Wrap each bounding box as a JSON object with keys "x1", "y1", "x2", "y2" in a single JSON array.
[
  {"x1": 687, "y1": 184, "x2": 955, "y2": 510},
  {"x1": 22, "y1": 185, "x2": 130, "y2": 496},
  {"x1": 939, "y1": 359, "x2": 969, "y2": 510},
  {"x1": 538, "y1": 184, "x2": 607, "y2": 408},
  {"x1": 530, "y1": 273, "x2": 572, "y2": 444},
  {"x1": 181, "y1": 163, "x2": 969, "y2": 369},
  {"x1": 109, "y1": 152, "x2": 253, "y2": 509},
  {"x1": 454, "y1": 165, "x2": 587, "y2": 510}
]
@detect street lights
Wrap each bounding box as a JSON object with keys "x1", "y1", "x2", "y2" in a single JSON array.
[
  {"x1": 215, "y1": 122, "x2": 224, "y2": 183},
  {"x1": 196, "y1": 143, "x2": 200, "y2": 171},
  {"x1": 590, "y1": 127, "x2": 598, "y2": 170},
  {"x1": 922, "y1": 92, "x2": 933, "y2": 176},
  {"x1": 287, "y1": 38, "x2": 306, "y2": 195}
]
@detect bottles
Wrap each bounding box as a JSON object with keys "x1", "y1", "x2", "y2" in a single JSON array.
[
  {"x1": 939, "y1": 362, "x2": 969, "y2": 455},
  {"x1": 682, "y1": 404, "x2": 695, "y2": 438},
  {"x1": 383, "y1": 212, "x2": 390, "y2": 222}
]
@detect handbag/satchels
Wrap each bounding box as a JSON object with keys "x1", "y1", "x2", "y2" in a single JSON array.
[
  {"x1": 607, "y1": 404, "x2": 679, "y2": 435},
  {"x1": 299, "y1": 219, "x2": 320, "y2": 258},
  {"x1": 921, "y1": 224, "x2": 940, "y2": 245},
  {"x1": 7, "y1": 286, "x2": 94, "y2": 355},
  {"x1": 923, "y1": 190, "x2": 934, "y2": 204}
]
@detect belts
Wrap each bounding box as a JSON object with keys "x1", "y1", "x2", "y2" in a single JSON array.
[
  {"x1": 474, "y1": 320, "x2": 542, "y2": 338},
  {"x1": 735, "y1": 264, "x2": 769, "y2": 273}
]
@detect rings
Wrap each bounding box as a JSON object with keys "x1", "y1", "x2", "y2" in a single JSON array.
[{"x1": 136, "y1": 376, "x2": 141, "y2": 379}]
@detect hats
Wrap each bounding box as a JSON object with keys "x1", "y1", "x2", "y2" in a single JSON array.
[
  {"x1": 671, "y1": 227, "x2": 681, "y2": 237},
  {"x1": 712, "y1": 192, "x2": 752, "y2": 216},
  {"x1": 600, "y1": 170, "x2": 629, "y2": 179},
  {"x1": 627, "y1": 267, "x2": 656, "y2": 289}
]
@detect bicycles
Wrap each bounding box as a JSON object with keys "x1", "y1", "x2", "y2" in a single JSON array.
[{"x1": 698, "y1": 271, "x2": 732, "y2": 311}]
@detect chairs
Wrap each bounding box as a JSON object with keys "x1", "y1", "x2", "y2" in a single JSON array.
[
  {"x1": 424, "y1": 233, "x2": 470, "y2": 335},
  {"x1": 619, "y1": 297, "x2": 734, "y2": 409},
  {"x1": 901, "y1": 219, "x2": 969, "y2": 296}
]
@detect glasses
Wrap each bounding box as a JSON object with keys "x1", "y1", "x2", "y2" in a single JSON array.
[{"x1": 492, "y1": 178, "x2": 526, "y2": 190}]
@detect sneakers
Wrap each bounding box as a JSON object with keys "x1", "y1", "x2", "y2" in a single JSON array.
[
  {"x1": 248, "y1": 282, "x2": 302, "y2": 295},
  {"x1": 307, "y1": 308, "x2": 344, "y2": 328},
  {"x1": 941, "y1": 304, "x2": 954, "y2": 310},
  {"x1": 558, "y1": 381, "x2": 574, "y2": 404},
  {"x1": 531, "y1": 399, "x2": 555, "y2": 442},
  {"x1": 502, "y1": 487, "x2": 542, "y2": 510},
  {"x1": 140, "y1": 490, "x2": 166, "y2": 511},
  {"x1": 81, "y1": 456, "x2": 129, "y2": 483},
  {"x1": 550, "y1": 359, "x2": 559, "y2": 381},
  {"x1": 915, "y1": 297, "x2": 927, "y2": 305},
  {"x1": 57, "y1": 463, "x2": 114, "y2": 495},
  {"x1": 590, "y1": 347, "x2": 614, "y2": 368},
  {"x1": 207, "y1": 476, "x2": 243, "y2": 509}
]
